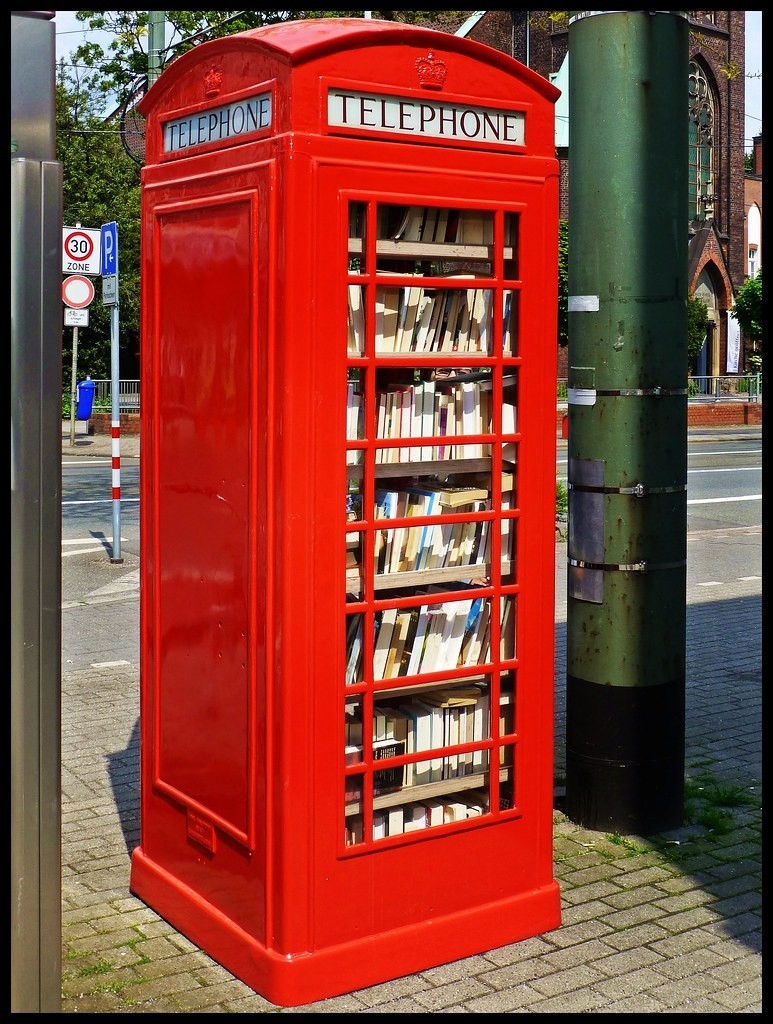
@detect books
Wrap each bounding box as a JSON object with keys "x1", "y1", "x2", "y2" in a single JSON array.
[{"x1": 346, "y1": 202, "x2": 517, "y2": 847}]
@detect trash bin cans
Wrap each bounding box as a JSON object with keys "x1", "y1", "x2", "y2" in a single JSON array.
[{"x1": 77, "y1": 380, "x2": 95, "y2": 420}]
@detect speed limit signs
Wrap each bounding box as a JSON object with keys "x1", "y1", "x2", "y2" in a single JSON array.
[{"x1": 64, "y1": 232, "x2": 94, "y2": 260}]
